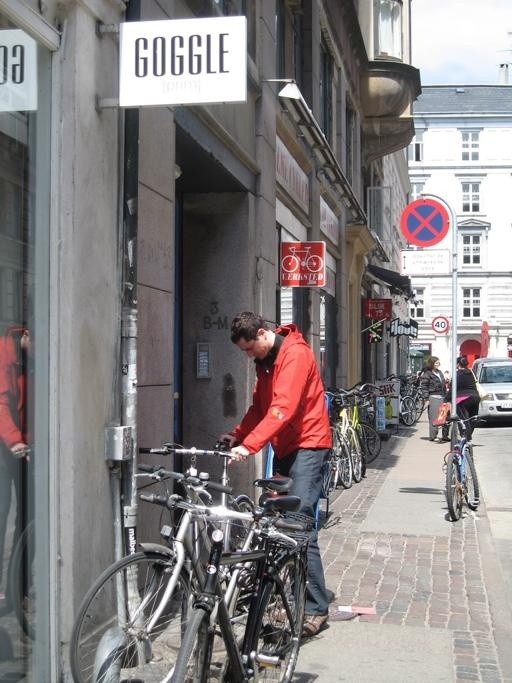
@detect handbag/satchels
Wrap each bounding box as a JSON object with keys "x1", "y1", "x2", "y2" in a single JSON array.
[{"x1": 431, "y1": 403, "x2": 450, "y2": 428}]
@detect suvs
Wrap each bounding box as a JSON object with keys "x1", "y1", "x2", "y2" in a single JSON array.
[{"x1": 471, "y1": 356, "x2": 511, "y2": 423}]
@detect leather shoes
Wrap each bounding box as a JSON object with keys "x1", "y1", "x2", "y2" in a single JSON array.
[
  {"x1": 300, "y1": 612, "x2": 328, "y2": 639},
  {"x1": 272, "y1": 605, "x2": 288, "y2": 622}
]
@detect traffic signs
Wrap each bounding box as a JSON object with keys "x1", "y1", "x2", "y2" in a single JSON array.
[{"x1": 431, "y1": 314, "x2": 449, "y2": 333}]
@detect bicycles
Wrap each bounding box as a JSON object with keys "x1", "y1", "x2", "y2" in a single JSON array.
[
  {"x1": 321, "y1": 367, "x2": 435, "y2": 496},
  {"x1": 70, "y1": 436, "x2": 308, "y2": 682},
  {"x1": 8, "y1": 445, "x2": 32, "y2": 640},
  {"x1": 432, "y1": 412, "x2": 491, "y2": 522}
]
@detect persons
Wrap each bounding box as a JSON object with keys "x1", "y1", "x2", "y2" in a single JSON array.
[
  {"x1": 218, "y1": 311, "x2": 334, "y2": 636},
  {"x1": 442, "y1": 356, "x2": 480, "y2": 434},
  {"x1": 1, "y1": 322, "x2": 33, "y2": 615},
  {"x1": 422, "y1": 356, "x2": 452, "y2": 441}
]
